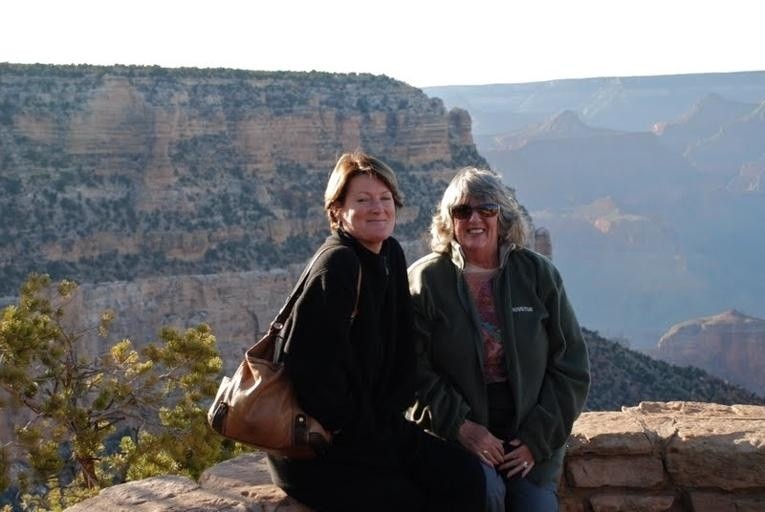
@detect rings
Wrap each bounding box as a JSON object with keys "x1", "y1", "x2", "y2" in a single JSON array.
[
  {"x1": 482, "y1": 450, "x2": 488, "y2": 454},
  {"x1": 523, "y1": 461, "x2": 528, "y2": 468}
]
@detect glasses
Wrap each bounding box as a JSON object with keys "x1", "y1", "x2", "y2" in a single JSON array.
[{"x1": 452, "y1": 203, "x2": 503, "y2": 219}]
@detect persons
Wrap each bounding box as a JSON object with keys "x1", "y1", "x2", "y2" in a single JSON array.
[
  {"x1": 264, "y1": 152, "x2": 487, "y2": 512},
  {"x1": 404, "y1": 166, "x2": 592, "y2": 512}
]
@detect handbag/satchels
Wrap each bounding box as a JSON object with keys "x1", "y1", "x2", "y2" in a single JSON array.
[{"x1": 206, "y1": 244, "x2": 364, "y2": 462}]
